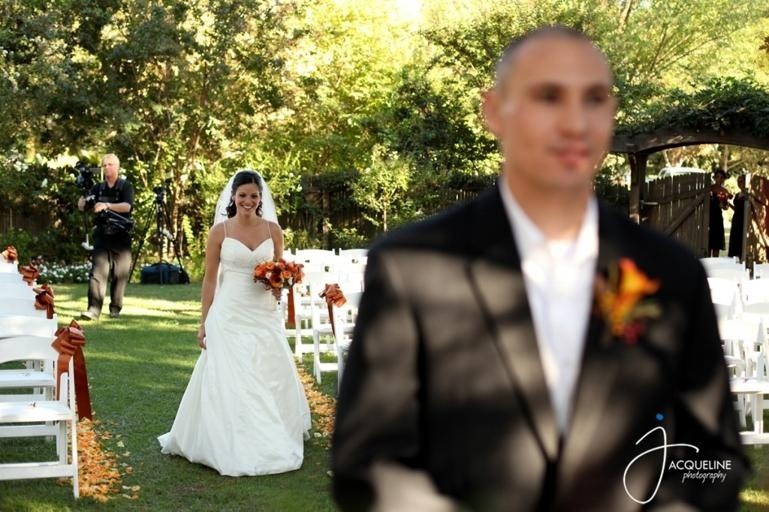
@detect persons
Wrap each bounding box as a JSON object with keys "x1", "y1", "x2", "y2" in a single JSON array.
[
  {"x1": 728, "y1": 174, "x2": 745, "y2": 257},
  {"x1": 330, "y1": 27, "x2": 754, "y2": 512},
  {"x1": 78, "y1": 153, "x2": 134, "y2": 320},
  {"x1": 157, "y1": 170, "x2": 313, "y2": 477},
  {"x1": 708, "y1": 168, "x2": 733, "y2": 257}
]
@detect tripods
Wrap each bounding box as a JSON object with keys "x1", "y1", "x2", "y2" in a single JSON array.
[{"x1": 128, "y1": 201, "x2": 188, "y2": 285}]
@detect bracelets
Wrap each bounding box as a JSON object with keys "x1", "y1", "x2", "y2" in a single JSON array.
[{"x1": 107, "y1": 203, "x2": 111, "y2": 209}]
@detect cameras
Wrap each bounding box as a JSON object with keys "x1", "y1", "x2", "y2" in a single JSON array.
[{"x1": 152, "y1": 186, "x2": 164, "y2": 201}]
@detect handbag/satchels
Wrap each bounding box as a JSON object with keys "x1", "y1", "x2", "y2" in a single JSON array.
[{"x1": 141, "y1": 260, "x2": 187, "y2": 284}]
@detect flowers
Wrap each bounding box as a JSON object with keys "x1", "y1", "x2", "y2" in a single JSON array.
[{"x1": 254, "y1": 260, "x2": 304, "y2": 300}]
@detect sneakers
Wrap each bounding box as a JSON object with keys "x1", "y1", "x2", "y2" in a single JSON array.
[
  {"x1": 111, "y1": 312, "x2": 119, "y2": 318},
  {"x1": 81, "y1": 309, "x2": 99, "y2": 321}
]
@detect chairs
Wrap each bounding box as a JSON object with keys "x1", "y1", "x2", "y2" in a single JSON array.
[
  {"x1": 0, "y1": 253, "x2": 80, "y2": 500},
  {"x1": 278, "y1": 249, "x2": 769, "y2": 444}
]
